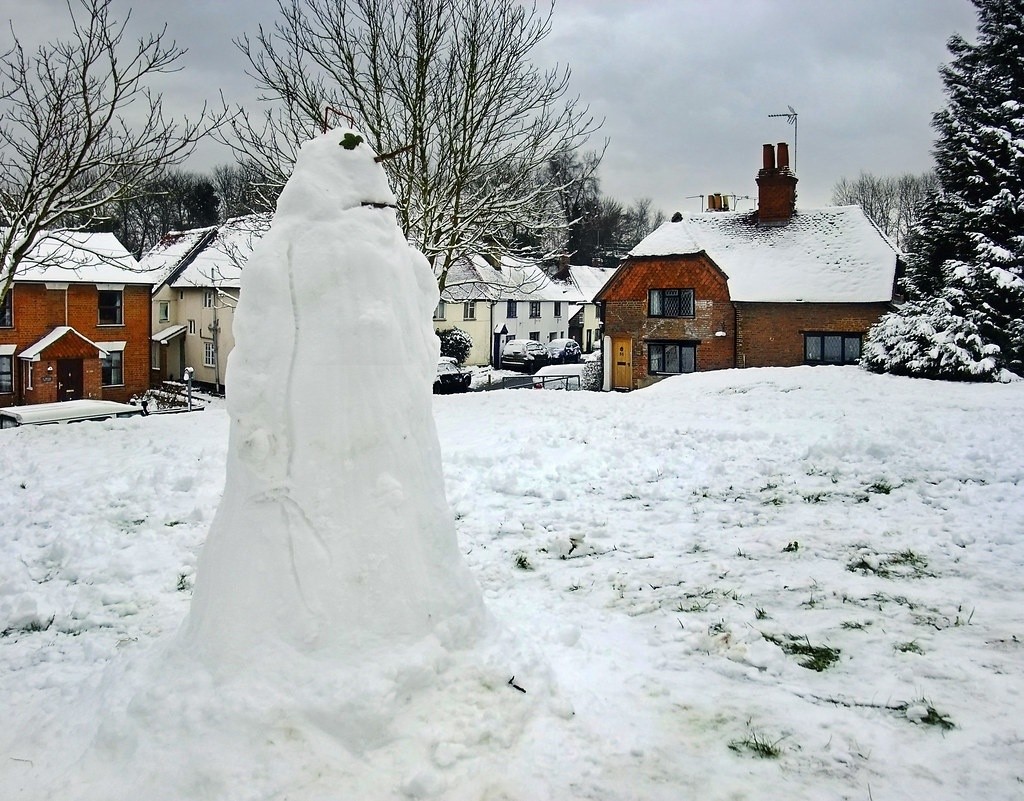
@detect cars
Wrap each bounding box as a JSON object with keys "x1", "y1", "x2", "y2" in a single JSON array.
[
  {"x1": 433, "y1": 362, "x2": 471, "y2": 393},
  {"x1": 547, "y1": 338, "x2": 581, "y2": 364},
  {"x1": 502, "y1": 339, "x2": 552, "y2": 375}
]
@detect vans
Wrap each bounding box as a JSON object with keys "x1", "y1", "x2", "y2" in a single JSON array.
[{"x1": 0, "y1": 399, "x2": 144, "y2": 430}]
